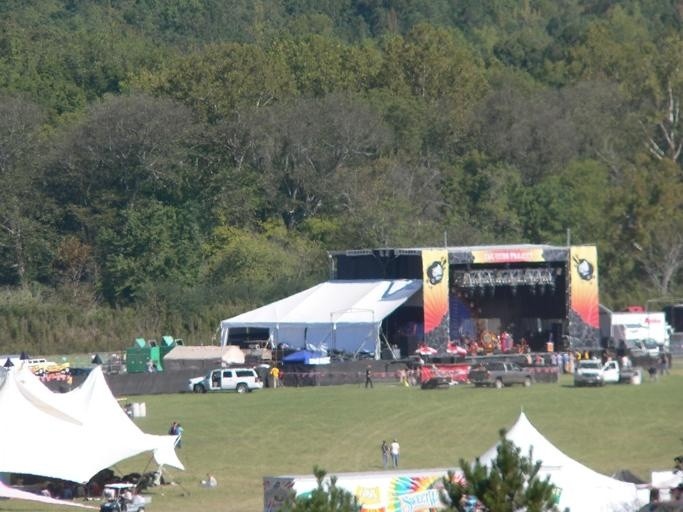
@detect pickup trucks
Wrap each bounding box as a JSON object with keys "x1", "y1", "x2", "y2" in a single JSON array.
[{"x1": 468, "y1": 360, "x2": 532, "y2": 389}]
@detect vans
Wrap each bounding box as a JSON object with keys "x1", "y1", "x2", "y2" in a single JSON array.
[
  {"x1": 187, "y1": 365, "x2": 264, "y2": 396},
  {"x1": 23, "y1": 358, "x2": 57, "y2": 376}
]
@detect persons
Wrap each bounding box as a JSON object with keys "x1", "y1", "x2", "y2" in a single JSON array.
[
  {"x1": 269, "y1": 364, "x2": 284, "y2": 390},
  {"x1": 381, "y1": 436, "x2": 402, "y2": 470},
  {"x1": 524, "y1": 348, "x2": 671, "y2": 382},
  {"x1": 169, "y1": 421, "x2": 184, "y2": 450},
  {"x1": 365, "y1": 364, "x2": 374, "y2": 389}
]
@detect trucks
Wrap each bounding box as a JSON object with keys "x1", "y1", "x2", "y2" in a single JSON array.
[{"x1": 598, "y1": 308, "x2": 669, "y2": 377}]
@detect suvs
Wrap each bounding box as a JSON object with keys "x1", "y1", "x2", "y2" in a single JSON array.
[{"x1": 573, "y1": 359, "x2": 621, "y2": 386}]
